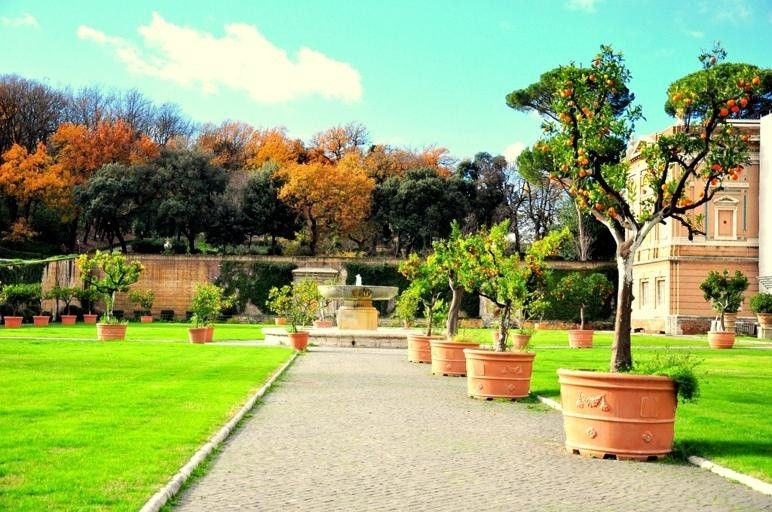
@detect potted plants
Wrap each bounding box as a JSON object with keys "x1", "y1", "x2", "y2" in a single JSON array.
[
  {"x1": 722, "y1": 293, "x2": 745, "y2": 333},
  {"x1": 749, "y1": 291, "x2": 772, "y2": 327},
  {"x1": 489, "y1": 287, "x2": 551, "y2": 348},
  {"x1": 698, "y1": 267, "x2": 749, "y2": 351},
  {"x1": 389, "y1": 288, "x2": 422, "y2": 330},
  {"x1": 553, "y1": 268, "x2": 616, "y2": 351},
  {"x1": 503, "y1": 39, "x2": 772, "y2": 466},
  {"x1": 429, "y1": 218, "x2": 503, "y2": 380},
  {"x1": 459, "y1": 227, "x2": 574, "y2": 402},
  {"x1": 390, "y1": 246, "x2": 445, "y2": 366},
  {"x1": 0, "y1": 248, "x2": 155, "y2": 341},
  {"x1": 263, "y1": 276, "x2": 333, "y2": 351},
  {"x1": 186, "y1": 281, "x2": 225, "y2": 344}
]
